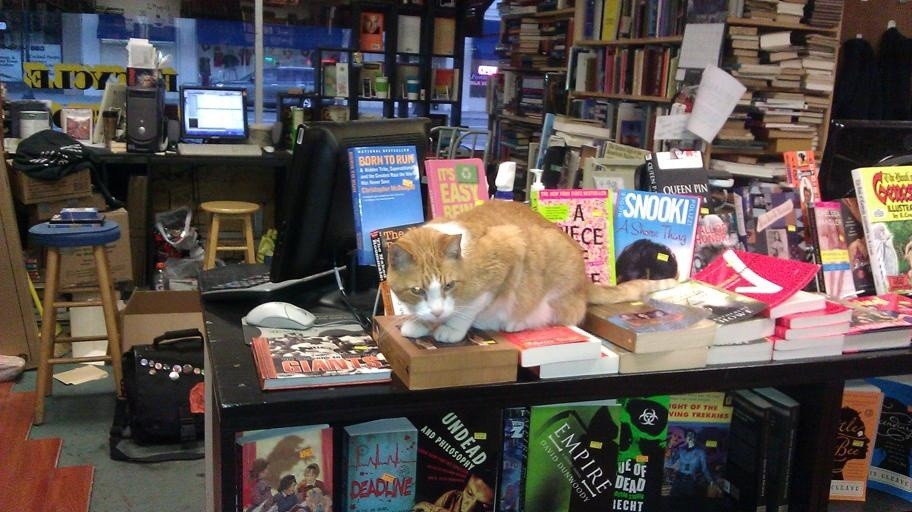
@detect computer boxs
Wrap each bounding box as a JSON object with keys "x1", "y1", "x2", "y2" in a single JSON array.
[{"x1": 125, "y1": 84, "x2": 167, "y2": 154}]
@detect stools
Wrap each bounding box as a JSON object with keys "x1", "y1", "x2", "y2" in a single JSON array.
[
  {"x1": 29, "y1": 220, "x2": 125, "y2": 426},
  {"x1": 200, "y1": 200, "x2": 260, "y2": 269}
]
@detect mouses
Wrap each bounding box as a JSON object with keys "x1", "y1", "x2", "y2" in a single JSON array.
[
  {"x1": 263, "y1": 145, "x2": 275, "y2": 153},
  {"x1": 245, "y1": 301, "x2": 317, "y2": 331}
]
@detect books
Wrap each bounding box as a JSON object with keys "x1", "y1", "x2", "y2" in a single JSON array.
[{"x1": 232, "y1": 1, "x2": 911, "y2": 512}]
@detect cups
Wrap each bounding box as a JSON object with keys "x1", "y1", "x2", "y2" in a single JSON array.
[
  {"x1": 375, "y1": 77, "x2": 390, "y2": 99},
  {"x1": 406, "y1": 76, "x2": 421, "y2": 100},
  {"x1": 102, "y1": 110, "x2": 119, "y2": 148}
]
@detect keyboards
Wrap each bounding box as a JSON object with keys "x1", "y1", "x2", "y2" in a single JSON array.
[
  {"x1": 201, "y1": 262, "x2": 346, "y2": 300},
  {"x1": 177, "y1": 142, "x2": 263, "y2": 157}
]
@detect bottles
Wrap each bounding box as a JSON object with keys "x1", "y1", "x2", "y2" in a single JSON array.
[{"x1": 152, "y1": 262, "x2": 170, "y2": 291}]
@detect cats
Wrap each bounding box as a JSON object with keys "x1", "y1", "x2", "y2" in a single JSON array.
[{"x1": 384, "y1": 200, "x2": 678, "y2": 344}]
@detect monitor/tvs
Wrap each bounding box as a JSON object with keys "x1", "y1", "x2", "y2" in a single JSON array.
[
  {"x1": 179, "y1": 85, "x2": 248, "y2": 143},
  {"x1": 269, "y1": 115, "x2": 432, "y2": 316}
]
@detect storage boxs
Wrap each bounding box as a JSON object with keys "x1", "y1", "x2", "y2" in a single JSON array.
[{"x1": 6, "y1": 157, "x2": 134, "y2": 291}]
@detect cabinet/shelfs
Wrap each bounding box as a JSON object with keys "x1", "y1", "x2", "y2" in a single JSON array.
[
  {"x1": 197, "y1": 259, "x2": 912, "y2": 512},
  {"x1": 311, "y1": 44, "x2": 464, "y2": 150}
]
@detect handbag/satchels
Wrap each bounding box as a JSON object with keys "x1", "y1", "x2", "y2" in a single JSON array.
[
  {"x1": 12, "y1": 129, "x2": 89, "y2": 183},
  {"x1": 154, "y1": 205, "x2": 206, "y2": 259},
  {"x1": 109, "y1": 328, "x2": 204, "y2": 464}
]
